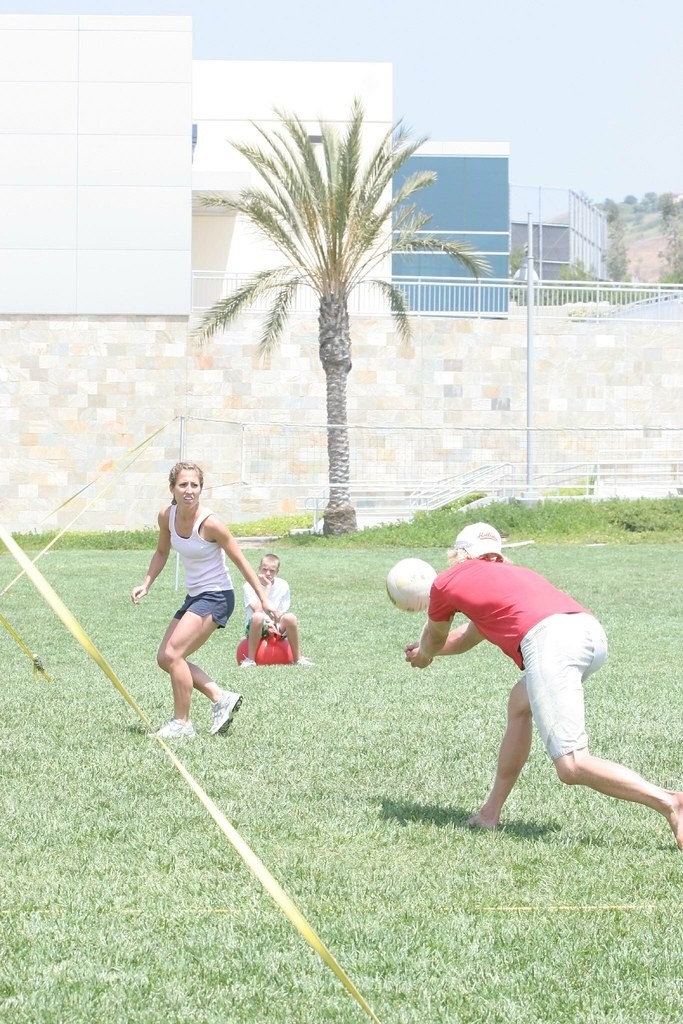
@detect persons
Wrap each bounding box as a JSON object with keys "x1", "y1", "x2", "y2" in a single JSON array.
[
  {"x1": 404, "y1": 522, "x2": 682, "y2": 849},
  {"x1": 239, "y1": 555, "x2": 316, "y2": 666},
  {"x1": 130, "y1": 462, "x2": 281, "y2": 739}
]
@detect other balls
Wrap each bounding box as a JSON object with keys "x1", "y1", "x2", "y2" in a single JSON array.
[{"x1": 237, "y1": 635, "x2": 294, "y2": 666}]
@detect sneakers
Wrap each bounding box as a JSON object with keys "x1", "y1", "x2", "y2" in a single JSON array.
[
  {"x1": 210, "y1": 690, "x2": 244, "y2": 735},
  {"x1": 148, "y1": 720, "x2": 196, "y2": 743}
]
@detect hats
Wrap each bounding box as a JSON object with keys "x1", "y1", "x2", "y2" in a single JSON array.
[{"x1": 456, "y1": 521, "x2": 504, "y2": 563}]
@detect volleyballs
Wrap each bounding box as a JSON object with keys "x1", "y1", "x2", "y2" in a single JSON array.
[{"x1": 385, "y1": 558, "x2": 439, "y2": 614}]
